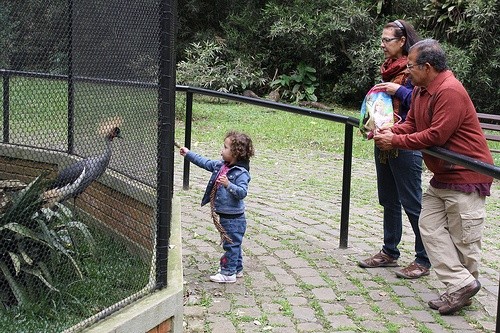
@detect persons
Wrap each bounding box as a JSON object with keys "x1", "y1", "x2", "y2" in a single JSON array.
[
  {"x1": 356, "y1": 19, "x2": 432, "y2": 280},
  {"x1": 372, "y1": 39, "x2": 495, "y2": 315},
  {"x1": 179, "y1": 129, "x2": 255, "y2": 283}
]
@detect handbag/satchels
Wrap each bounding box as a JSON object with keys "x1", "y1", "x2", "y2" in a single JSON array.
[{"x1": 358, "y1": 80, "x2": 401, "y2": 140}]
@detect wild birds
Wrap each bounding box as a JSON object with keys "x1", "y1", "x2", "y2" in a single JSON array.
[{"x1": 0, "y1": 126, "x2": 125, "y2": 216}]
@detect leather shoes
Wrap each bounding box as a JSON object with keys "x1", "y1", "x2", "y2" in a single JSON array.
[
  {"x1": 439, "y1": 280, "x2": 482, "y2": 313},
  {"x1": 428, "y1": 291, "x2": 473, "y2": 310},
  {"x1": 358, "y1": 250, "x2": 397, "y2": 267},
  {"x1": 394, "y1": 260, "x2": 430, "y2": 279}
]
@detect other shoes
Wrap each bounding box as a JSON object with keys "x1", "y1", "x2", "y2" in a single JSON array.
[
  {"x1": 210, "y1": 272, "x2": 236, "y2": 282},
  {"x1": 218, "y1": 267, "x2": 243, "y2": 277}
]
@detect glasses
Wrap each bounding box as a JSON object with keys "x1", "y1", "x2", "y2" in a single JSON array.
[
  {"x1": 381, "y1": 37, "x2": 400, "y2": 42},
  {"x1": 406, "y1": 62, "x2": 425, "y2": 70}
]
